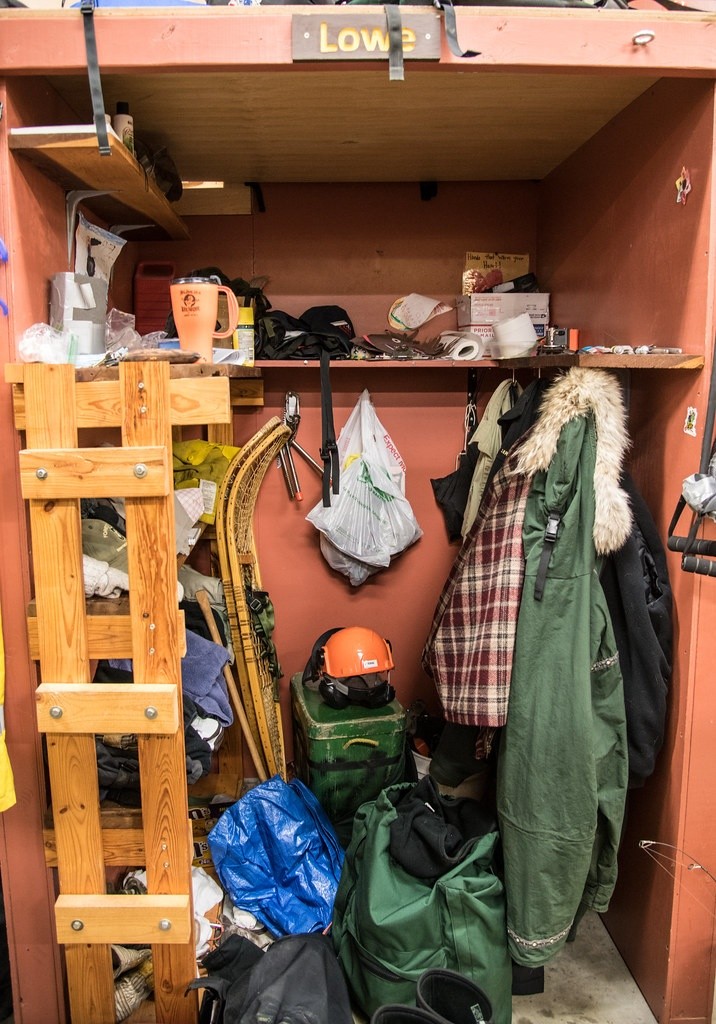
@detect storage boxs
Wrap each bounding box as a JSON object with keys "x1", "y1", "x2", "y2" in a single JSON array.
[
  {"x1": 455, "y1": 292, "x2": 551, "y2": 356},
  {"x1": 287, "y1": 671, "x2": 408, "y2": 822}
]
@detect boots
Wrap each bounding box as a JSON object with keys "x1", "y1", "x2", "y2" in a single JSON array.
[{"x1": 371, "y1": 968, "x2": 494, "y2": 1024}]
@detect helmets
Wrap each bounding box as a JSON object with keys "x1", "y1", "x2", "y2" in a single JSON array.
[{"x1": 302, "y1": 626, "x2": 397, "y2": 710}]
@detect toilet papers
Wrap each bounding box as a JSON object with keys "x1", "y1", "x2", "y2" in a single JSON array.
[
  {"x1": 439, "y1": 335, "x2": 480, "y2": 361},
  {"x1": 491, "y1": 311, "x2": 537, "y2": 359}
]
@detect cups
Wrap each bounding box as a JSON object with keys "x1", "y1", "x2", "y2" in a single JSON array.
[{"x1": 170, "y1": 277, "x2": 239, "y2": 365}]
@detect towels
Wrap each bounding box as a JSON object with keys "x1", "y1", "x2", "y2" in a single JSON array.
[
  {"x1": 81, "y1": 552, "x2": 184, "y2": 604},
  {"x1": 108, "y1": 627, "x2": 236, "y2": 727}
]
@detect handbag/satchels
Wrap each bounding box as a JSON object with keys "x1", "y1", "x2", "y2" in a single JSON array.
[
  {"x1": 188, "y1": 932, "x2": 353, "y2": 1024},
  {"x1": 329, "y1": 782, "x2": 513, "y2": 1024},
  {"x1": 304, "y1": 390, "x2": 423, "y2": 590},
  {"x1": 207, "y1": 775, "x2": 348, "y2": 940}
]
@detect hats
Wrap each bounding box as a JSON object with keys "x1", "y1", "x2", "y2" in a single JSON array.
[{"x1": 298, "y1": 305, "x2": 357, "y2": 354}]
